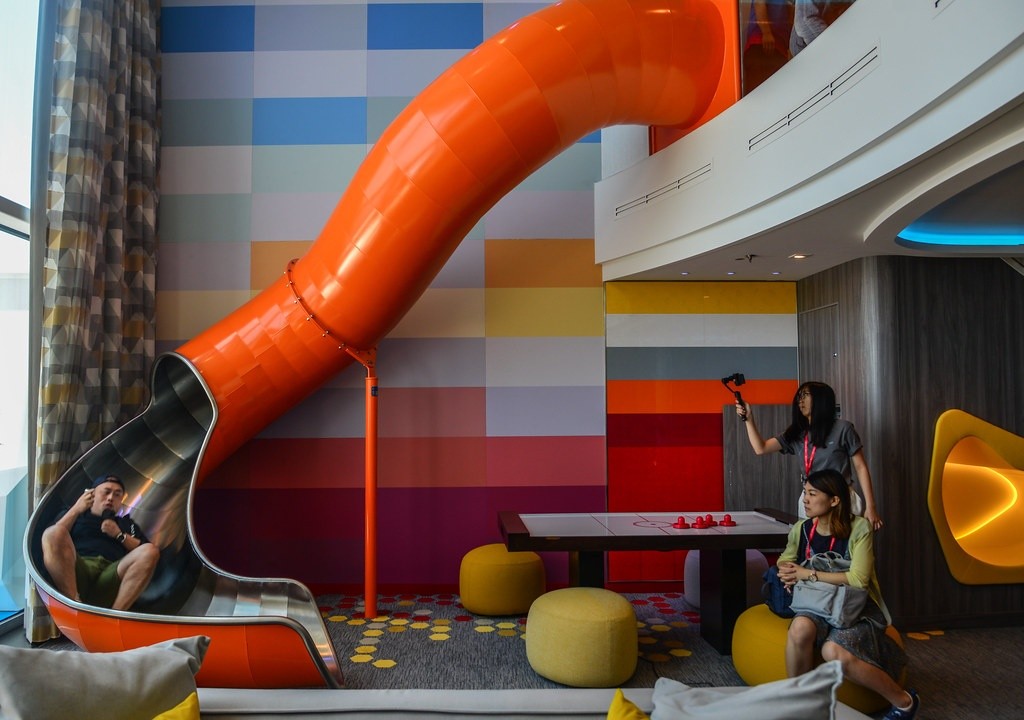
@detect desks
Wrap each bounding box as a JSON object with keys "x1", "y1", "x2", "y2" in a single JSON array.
[{"x1": 498, "y1": 507, "x2": 806, "y2": 656}]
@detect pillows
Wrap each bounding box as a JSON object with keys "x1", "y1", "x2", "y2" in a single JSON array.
[
  {"x1": 651, "y1": 659, "x2": 844, "y2": 719},
  {"x1": 605, "y1": 688, "x2": 650, "y2": 720},
  {"x1": 0, "y1": 634, "x2": 211, "y2": 719}
]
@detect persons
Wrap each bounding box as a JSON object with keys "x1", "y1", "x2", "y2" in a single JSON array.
[
  {"x1": 776, "y1": 470, "x2": 921, "y2": 720},
  {"x1": 735, "y1": 382, "x2": 892, "y2": 626},
  {"x1": 42, "y1": 476, "x2": 159, "y2": 612}
]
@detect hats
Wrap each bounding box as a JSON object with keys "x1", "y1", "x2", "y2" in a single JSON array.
[{"x1": 89, "y1": 474, "x2": 125, "y2": 494}]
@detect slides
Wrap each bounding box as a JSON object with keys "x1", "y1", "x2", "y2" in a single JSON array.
[{"x1": 16, "y1": 1, "x2": 726, "y2": 690}]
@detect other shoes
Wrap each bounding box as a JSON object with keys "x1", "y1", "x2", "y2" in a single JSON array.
[{"x1": 883, "y1": 689, "x2": 920, "y2": 720}]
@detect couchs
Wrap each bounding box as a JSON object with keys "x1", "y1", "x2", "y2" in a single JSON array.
[{"x1": 196, "y1": 688, "x2": 877, "y2": 720}]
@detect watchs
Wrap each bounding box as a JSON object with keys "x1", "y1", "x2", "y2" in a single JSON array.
[
  {"x1": 116, "y1": 532, "x2": 126, "y2": 543},
  {"x1": 808, "y1": 570, "x2": 818, "y2": 583}
]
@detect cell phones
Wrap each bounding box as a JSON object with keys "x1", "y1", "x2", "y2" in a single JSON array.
[{"x1": 733, "y1": 374, "x2": 747, "y2": 386}]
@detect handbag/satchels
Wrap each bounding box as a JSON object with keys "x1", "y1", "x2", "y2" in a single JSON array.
[
  {"x1": 765, "y1": 567, "x2": 794, "y2": 619},
  {"x1": 789, "y1": 551, "x2": 868, "y2": 629}
]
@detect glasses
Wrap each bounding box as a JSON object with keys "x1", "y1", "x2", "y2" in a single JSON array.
[{"x1": 796, "y1": 392, "x2": 811, "y2": 401}]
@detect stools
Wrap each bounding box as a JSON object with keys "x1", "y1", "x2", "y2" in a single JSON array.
[
  {"x1": 684, "y1": 549, "x2": 769, "y2": 610},
  {"x1": 732, "y1": 604, "x2": 795, "y2": 686},
  {"x1": 813, "y1": 623, "x2": 907, "y2": 715},
  {"x1": 458, "y1": 543, "x2": 546, "y2": 616},
  {"x1": 526, "y1": 587, "x2": 639, "y2": 688}
]
